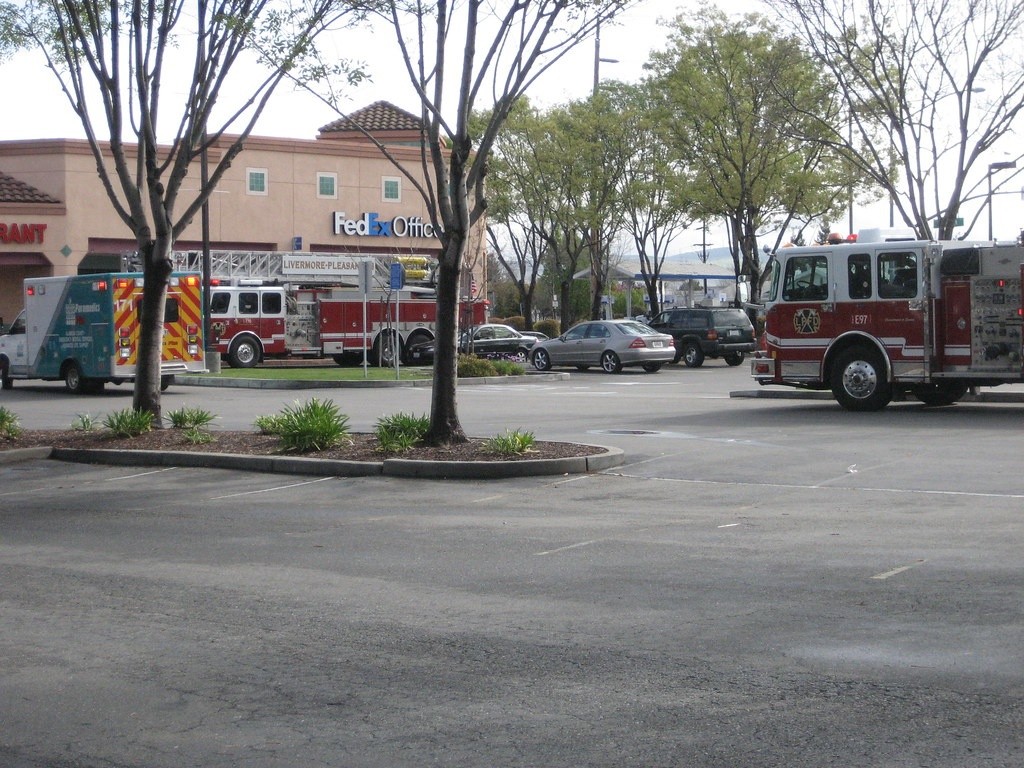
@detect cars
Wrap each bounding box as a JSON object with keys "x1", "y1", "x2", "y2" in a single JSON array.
[
  {"x1": 530, "y1": 319, "x2": 676, "y2": 375},
  {"x1": 408, "y1": 324, "x2": 541, "y2": 365},
  {"x1": 517, "y1": 330, "x2": 552, "y2": 343}
]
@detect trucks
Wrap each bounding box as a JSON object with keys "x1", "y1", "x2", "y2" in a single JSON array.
[{"x1": 0, "y1": 271, "x2": 211, "y2": 393}]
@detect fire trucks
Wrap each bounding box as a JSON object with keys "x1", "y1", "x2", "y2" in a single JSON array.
[
  {"x1": 748, "y1": 225, "x2": 1023, "y2": 412},
  {"x1": 199, "y1": 253, "x2": 491, "y2": 370}
]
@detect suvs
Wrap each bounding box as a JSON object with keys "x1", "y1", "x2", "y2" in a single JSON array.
[{"x1": 641, "y1": 307, "x2": 760, "y2": 366}]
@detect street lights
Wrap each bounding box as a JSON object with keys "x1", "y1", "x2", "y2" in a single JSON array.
[
  {"x1": 692, "y1": 219, "x2": 715, "y2": 297},
  {"x1": 988, "y1": 161, "x2": 1023, "y2": 243},
  {"x1": 589, "y1": 11, "x2": 622, "y2": 313},
  {"x1": 888, "y1": 86, "x2": 987, "y2": 231}
]
